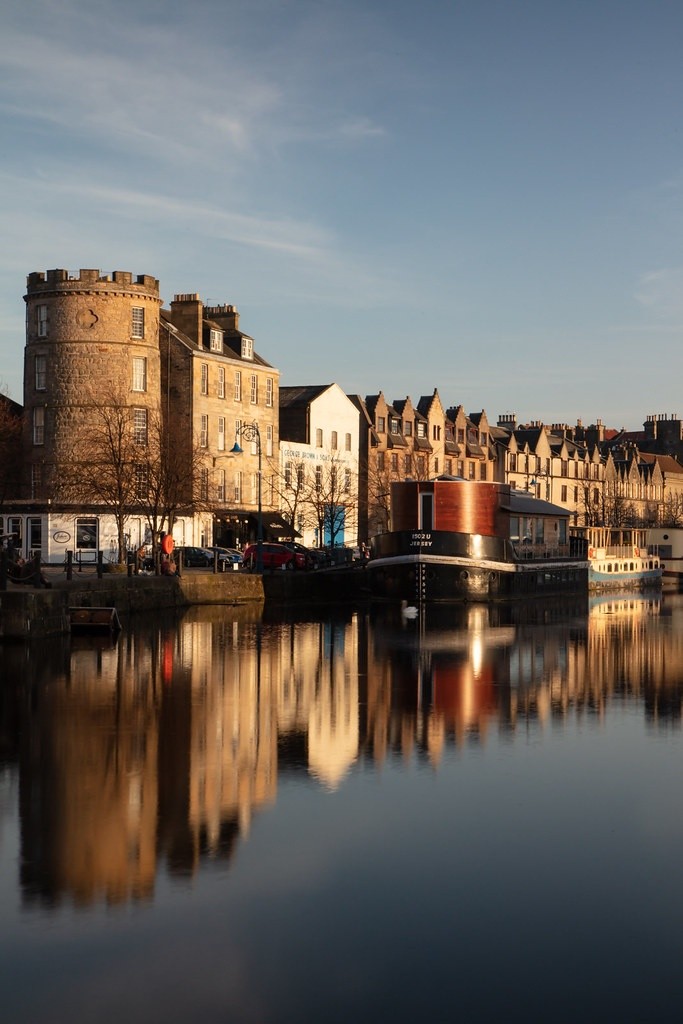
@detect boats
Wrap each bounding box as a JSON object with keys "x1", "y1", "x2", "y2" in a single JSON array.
[{"x1": 587, "y1": 526, "x2": 663, "y2": 582}]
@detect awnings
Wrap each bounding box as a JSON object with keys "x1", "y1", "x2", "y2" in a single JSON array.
[{"x1": 252, "y1": 511, "x2": 303, "y2": 538}]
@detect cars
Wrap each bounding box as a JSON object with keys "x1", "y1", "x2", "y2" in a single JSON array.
[
  {"x1": 244, "y1": 541, "x2": 327, "y2": 570},
  {"x1": 172, "y1": 546, "x2": 245, "y2": 568}
]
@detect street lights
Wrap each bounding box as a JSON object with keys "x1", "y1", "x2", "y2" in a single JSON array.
[{"x1": 230, "y1": 424, "x2": 265, "y2": 573}]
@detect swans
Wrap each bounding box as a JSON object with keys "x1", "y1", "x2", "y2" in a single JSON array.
[{"x1": 400, "y1": 600, "x2": 418, "y2": 614}]
